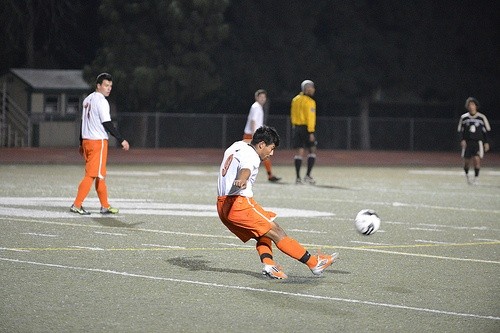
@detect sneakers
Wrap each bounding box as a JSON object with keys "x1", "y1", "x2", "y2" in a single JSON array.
[
  {"x1": 309, "y1": 251, "x2": 340, "y2": 275},
  {"x1": 262, "y1": 263, "x2": 288, "y2": 280}
]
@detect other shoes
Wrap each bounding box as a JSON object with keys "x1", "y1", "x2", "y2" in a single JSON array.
[
  {"x1": 268, "y1": 176, "x2": 282, "y2": 181},
  {"x1": 70, "y1": 204, "x2": 91, "y2": 215},
  {"x1": 100, "y1": 205, "x2": 119, "y2": 214}
]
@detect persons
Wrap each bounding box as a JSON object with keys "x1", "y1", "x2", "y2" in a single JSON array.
[
  {"x1": 70, "y1": 73, "x2": 130, "y2": 216},
  {"x1": 290, "y1": 80, "x2": 319, "y2": 185},
  {"x1": 457, "y1": 97, "x2": 491, "y2": 180},
  {"x1": 243, "y1": 88, "x2": 282, "y2": 182},
  {"x1": 218, "y1": 125, "x2": 340, "y2": 280}
]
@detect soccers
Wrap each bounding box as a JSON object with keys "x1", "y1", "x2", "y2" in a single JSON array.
[{"x1": 355, "y1": 208, "x2": 382, "y2": 238}]
